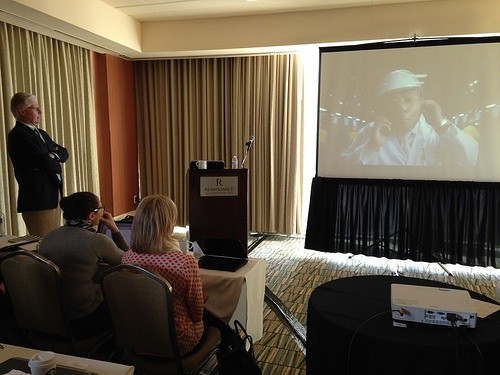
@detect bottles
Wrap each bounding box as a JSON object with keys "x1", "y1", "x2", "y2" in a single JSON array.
[
  {"x1": 232, "y1": 156, "x2": 238, "y2": 169},
  {"x1": 0, "y1": 209, "x2": 5, "y2": 237}
]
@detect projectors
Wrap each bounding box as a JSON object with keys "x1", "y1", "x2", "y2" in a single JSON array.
[{"x1": 390, "y1": 284, "x2": 478, "y2": 329}]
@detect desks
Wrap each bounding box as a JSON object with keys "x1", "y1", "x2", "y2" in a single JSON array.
[
  {"x1": 305, "y1": 275, "x2": 500, "y2": 375},
  {"x1": 0, "y1": 343, "x2": 134, "y2": 375},
  {"x1": 187, "y1": 250, "x2": 267, "y2": 353}
]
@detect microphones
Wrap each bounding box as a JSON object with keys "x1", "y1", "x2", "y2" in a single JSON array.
[{"x1": 250, "y1": 136, "x2": 255, "y2": 142}]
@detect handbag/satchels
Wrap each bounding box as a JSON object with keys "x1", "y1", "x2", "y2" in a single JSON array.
[{"x1": 216, "y1": 320, "x2": 262, "y2": 375}]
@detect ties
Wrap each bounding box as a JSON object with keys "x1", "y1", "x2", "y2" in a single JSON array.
[{"x1": 34, "y1": 129, "x2": 40, "y2": 135}]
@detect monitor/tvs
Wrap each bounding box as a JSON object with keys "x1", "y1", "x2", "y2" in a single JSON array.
[{"x1": 190, "y1": 161, "x2": 224, "y2": 169}]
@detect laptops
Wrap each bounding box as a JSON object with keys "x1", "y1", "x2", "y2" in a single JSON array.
[
  {"x1": 96, "y1": 220, "x2": 133, "y2": 249},
  {"x1": 194, "y1": 237, "x2": 249, "y2": 272}
]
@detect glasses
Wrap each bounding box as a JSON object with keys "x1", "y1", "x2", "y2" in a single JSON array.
[{"x1": 92, "y1": 206, "x2": 105, "y2": 212}]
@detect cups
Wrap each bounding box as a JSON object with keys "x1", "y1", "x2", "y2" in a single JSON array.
[
  {"x1": 179, "y1": 239, "x2": 193, "y2": 254},
  {"x1": 28, "y1": 351, "x2": 57, "y2": 375},
  {"x1": 196, "y1": 160, "x2": 207, "y2": 169}
]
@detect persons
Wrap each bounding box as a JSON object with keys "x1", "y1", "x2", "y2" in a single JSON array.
[
  {"x1": 320, "y1": 70, "x2": 480, "y2": 170},
  {"x1": 7, "y1": 93, "x2": 69, "y2": 237},
  {"x1": 39, "y1": 192, "x2": 130, "y2": 341},
  {"x1": 122, "y1": 195, "x2": 242, "y2": 359}
]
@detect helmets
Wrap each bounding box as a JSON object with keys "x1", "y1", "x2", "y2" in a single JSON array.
[{"x1": 379, "y1": 70, "x2": 421, "y2": 95}]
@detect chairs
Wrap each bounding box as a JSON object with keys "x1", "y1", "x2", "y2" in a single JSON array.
[{"x1": 0, "y1": 249, "x2": 222, "y2": 375}]
